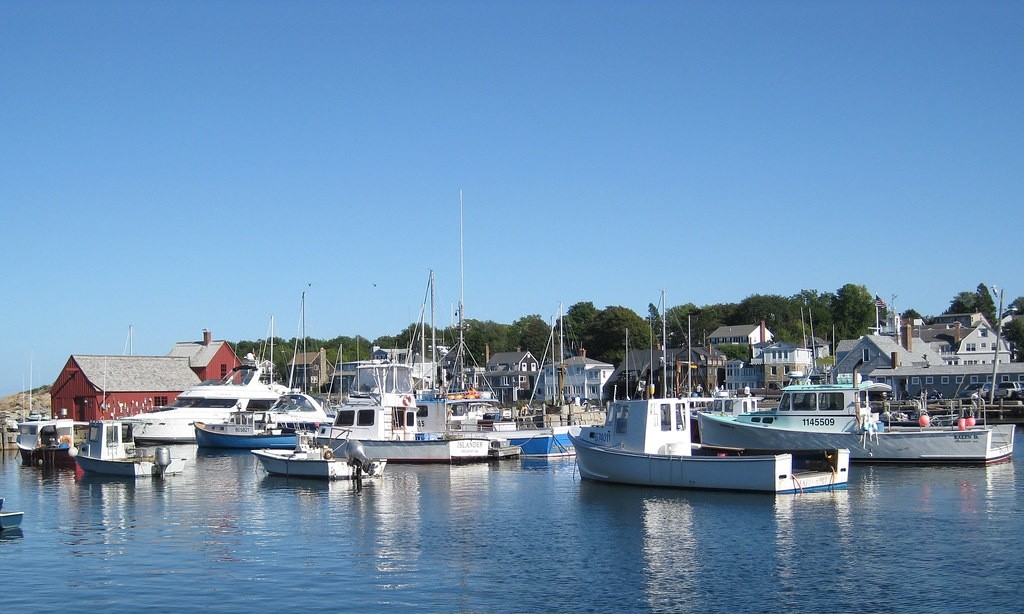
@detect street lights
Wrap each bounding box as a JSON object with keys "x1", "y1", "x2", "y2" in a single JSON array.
[{"x1": 989, "y1": 288, "x2": 1018, "y2": 405}]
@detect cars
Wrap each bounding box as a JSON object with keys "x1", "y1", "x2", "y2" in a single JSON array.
[{"x1": 956, "y1": 382, "x2": 999, "y2": 404}]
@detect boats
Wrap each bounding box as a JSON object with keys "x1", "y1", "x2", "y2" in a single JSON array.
[
  {"x1": 0, "y1": 497, "x2": 25, "y2": 531},
  {"x1": 14, "y1": 268, "x2": 1024, "y2": 494}
]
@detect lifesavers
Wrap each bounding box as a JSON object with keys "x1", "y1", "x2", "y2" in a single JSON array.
[
  {"x1": 324, "y1": 450, "x2": 332, "y2": 459},
  {"x1": 61, "y1": 436, "x2": 71, "y2": 446},
  {"x1": 403, "y1": 395, "x2": 411, "y2": 406}
]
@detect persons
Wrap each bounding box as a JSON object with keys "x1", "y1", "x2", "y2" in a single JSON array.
[
  {"x1": 566, "y1": 395, "x2": 580, "y2": 406},
  {"x1": 696, "y1": 383, "x2": 703, "y2": 397}
]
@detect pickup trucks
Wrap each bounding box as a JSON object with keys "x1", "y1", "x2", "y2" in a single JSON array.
[{"x1": 989, "y1": 381, "x2": 1024, "y2": 398}]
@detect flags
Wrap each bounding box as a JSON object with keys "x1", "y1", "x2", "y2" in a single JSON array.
[{"x1": 875, "y1": 295, "x2": 887, "y2": 309}]
